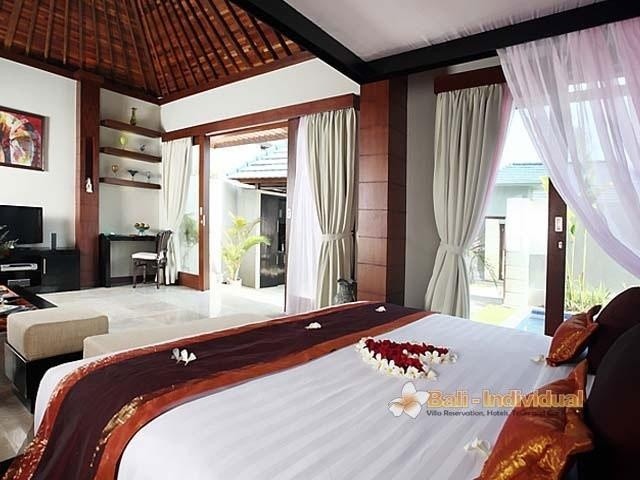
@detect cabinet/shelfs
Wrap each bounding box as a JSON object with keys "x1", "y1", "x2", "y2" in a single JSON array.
[
  {"x1": 99, "y1": 119, "x2": 162, "y2": 190},
  {"x1": 0, "y1": 247, "x2": 80, "y2": 293}
]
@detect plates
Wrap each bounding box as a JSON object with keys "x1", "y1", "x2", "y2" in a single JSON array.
[{"x1": 134, "y1": 227, "x2": 150, "y2": 236}]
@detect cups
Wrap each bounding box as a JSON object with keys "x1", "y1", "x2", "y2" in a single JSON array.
[
  {"x1": 111, "y1": 164, "x2": 119, "y2": 177},
  {"x1": 128, "y1": 170, "x2": 139, "y2": 181}
]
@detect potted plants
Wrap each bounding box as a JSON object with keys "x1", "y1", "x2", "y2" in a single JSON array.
[{"x1": 222, "y1": 208, "x2": 272, "y2": 286}]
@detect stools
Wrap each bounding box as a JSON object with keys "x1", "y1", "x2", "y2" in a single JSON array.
[{"x1": 5, "y1": 303, "x2": 109, "y2": 413}]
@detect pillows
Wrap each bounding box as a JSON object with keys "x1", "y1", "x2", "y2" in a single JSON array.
[
  {"x1": 546, "y1": 305, "x2": 603, "y2": 367},
  {"x1": 578, "y1": 325, "x2": 640, "y2": 480},
  {"x1": 589, "y1": 286, "x2": 640, "y2": 372},
  {"x1": 472, "y1": 358, "x2": 593, "y2": 480}
]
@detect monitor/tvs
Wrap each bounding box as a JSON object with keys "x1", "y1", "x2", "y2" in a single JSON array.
[{"x1": 1, "y1": 205, "x2": 44, "y2": 246}]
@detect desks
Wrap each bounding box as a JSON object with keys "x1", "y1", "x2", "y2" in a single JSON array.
[{"x1": 99, "y1": 233, "x2": 160, "y2": 288}]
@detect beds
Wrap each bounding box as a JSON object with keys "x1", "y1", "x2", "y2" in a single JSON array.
[{"x1": 1, "y1": 298, "x2": 595, "y2": 480}]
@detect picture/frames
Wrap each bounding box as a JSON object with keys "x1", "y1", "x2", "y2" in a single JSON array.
[{"x1": 0, "y1": 106, "x2": 45, "y2": 172}]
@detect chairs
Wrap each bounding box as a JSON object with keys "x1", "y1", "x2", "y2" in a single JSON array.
[{"x1": 130, "y1": 230, "x2": 172, "y2": 289}]
